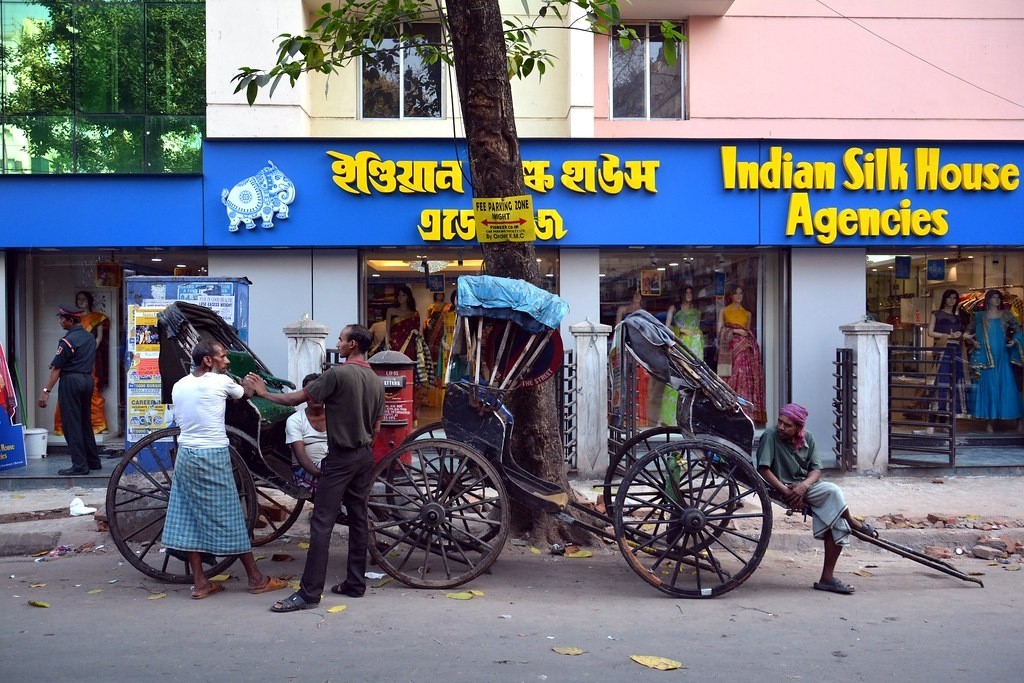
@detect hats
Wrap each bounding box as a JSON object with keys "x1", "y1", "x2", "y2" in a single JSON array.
[{"x1": 56, "y1": 305, "x2": 84, "y2": 317}]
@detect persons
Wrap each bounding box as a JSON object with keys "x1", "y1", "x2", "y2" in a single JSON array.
[
  {"x1": 38, "y1": 305, "x2": 102, "y2": 476},
  {"x1": 380, "y1": 284, "x2": 459, "y2": 409},
  {"x1": 717, "y1": 285, "x2": 762, "y2": 419},
  {"x1": 368, "y1": 318, "x2": 386, "y2": 351},
  {"x1": 161, "y1": 338, "x2": 289, "y2": 600},
  {"x1": 246, "y1": 324, "x2": 387, "y2": 612},
  {"x1": 757, "y1": 403, "x2": 879, "y2": 594},
  {"x1": 965, "y1": 289, "x2": 1023, "y2": 435},
  {"x1": 51, "y1": 291, "x2": 111, "y2": 435},
  {"x1": 613, "y1": 284, "x2": 650, "y2": 429},
  {"x1": 664, "y1": 284, "x2": 704, "y2": 426},
  {"x1": 921, "y1": 288, "x2": 966, "y2": 434},
  {"x1": 285, "y1": 373, "x2": 392, "y2": 563}
]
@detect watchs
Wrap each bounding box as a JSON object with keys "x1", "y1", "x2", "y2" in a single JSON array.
[{"x1": 42, "y1": 387, "x2": 50, "y2": 394}]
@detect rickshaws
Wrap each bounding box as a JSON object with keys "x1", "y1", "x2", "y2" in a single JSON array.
[
  {"x1": 349, "y1": 273, "x2": 735, "y2": 592},
  {"x1": 105, "y1": 295, "x2": 500, "y2": 586},
  {"x1": 597, "y1": 306, "x2": 986, "y2": 597}
]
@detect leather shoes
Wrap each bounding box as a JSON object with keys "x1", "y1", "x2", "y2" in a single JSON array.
[
  {"x1": 58, "y1": 466, "x2": 89, "y2": 476},
  {"x1": 88, "y1": 464, "x2": 102, "y2": 471}
]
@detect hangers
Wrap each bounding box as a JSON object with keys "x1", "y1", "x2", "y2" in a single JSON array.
[{"x1": 958, "y1": 284, "x2": 1024, "y2": 311}]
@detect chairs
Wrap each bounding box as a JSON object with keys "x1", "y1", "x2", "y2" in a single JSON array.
[
  {"x1": 221, "y1": 349, "x2": 296, "y2": 456},
  {"x1": 676, "y1": 383, "x2": 756, "y2": 486},
  {"x1": 442, "y1": 380, "x2": 515, "y2": 489}
]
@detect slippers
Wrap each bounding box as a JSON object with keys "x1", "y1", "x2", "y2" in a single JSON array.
[
  {"x1": 270, "y1": 591, "x2": 320, "y2": 612},
  {"x1": 249, "y1": 576, "x2": 288, "y2": 594},
  {"x1": 331, "y1": 584, "x2": 346, "y2": 595},
  {"x1": 192, "y1": 581, "x2": 225, "y2": 599},
  {"x1": 856, "y1": 522, "x2": 879, "y2": 540},
  {"x1": 814, "y1": 578, "x2": 855, "y2": 593}
]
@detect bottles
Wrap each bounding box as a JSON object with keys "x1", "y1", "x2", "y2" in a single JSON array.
[{"x1": 665, "y1": 451, "x2": 680, "y2": 502}]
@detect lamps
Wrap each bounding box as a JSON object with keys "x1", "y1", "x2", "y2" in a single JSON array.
[
  {"x1": 648, "y1": 252, "x2": 725, "y2": 266},
  {"x1": 421, "y1": 249, "x2": 465, "y2": 267}
]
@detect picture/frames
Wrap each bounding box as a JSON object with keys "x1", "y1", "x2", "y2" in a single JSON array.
[
  {"x1": 640, "y1": 270, "x2": 662, "y2": 296},
  {"x1": 428, "y1": 274, "x2": 446, "y2": 293},
  {"x1": 174, "y1": 268, "x2": 194, "y2": 276}
]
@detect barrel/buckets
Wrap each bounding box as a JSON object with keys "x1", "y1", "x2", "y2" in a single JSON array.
[{"x1": 25, "y1": 428, "x2": 49, "y2": 459}]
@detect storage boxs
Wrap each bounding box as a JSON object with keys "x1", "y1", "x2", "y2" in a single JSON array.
[{"x1": 367, "y1": 285, "x2": 396, "y2": 322}]
